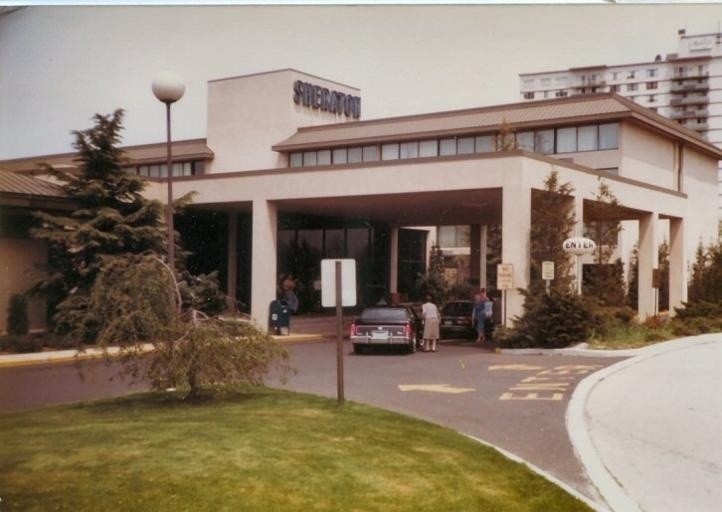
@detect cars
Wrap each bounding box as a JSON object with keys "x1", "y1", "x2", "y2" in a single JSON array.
[
  {"x1": 349, "y1": 304, "x2": 421, "y2": 353},
  {"x1": 438, "y1": 301, "x2": 495, "y2": 340}
]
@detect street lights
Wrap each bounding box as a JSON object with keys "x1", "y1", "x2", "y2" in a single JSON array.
[{"x1": 152, "y1": 70, "x2": 186, "y2": 389}]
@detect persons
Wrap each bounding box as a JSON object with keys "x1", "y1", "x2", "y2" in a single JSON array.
[
  {"x1": 417, "y1": 293, "x2": 442, "y2": 353},
  {"x1": 460, "y1": 285, "x2": 498, "y2": 346},
  {"x1": 281, "y1": 272, "x2": 299, "y2": 314}
]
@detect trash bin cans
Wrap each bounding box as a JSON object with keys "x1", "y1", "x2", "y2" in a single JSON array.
[{"x1": 270, "y1": 300, "x2": 290, "y2": 336}]
